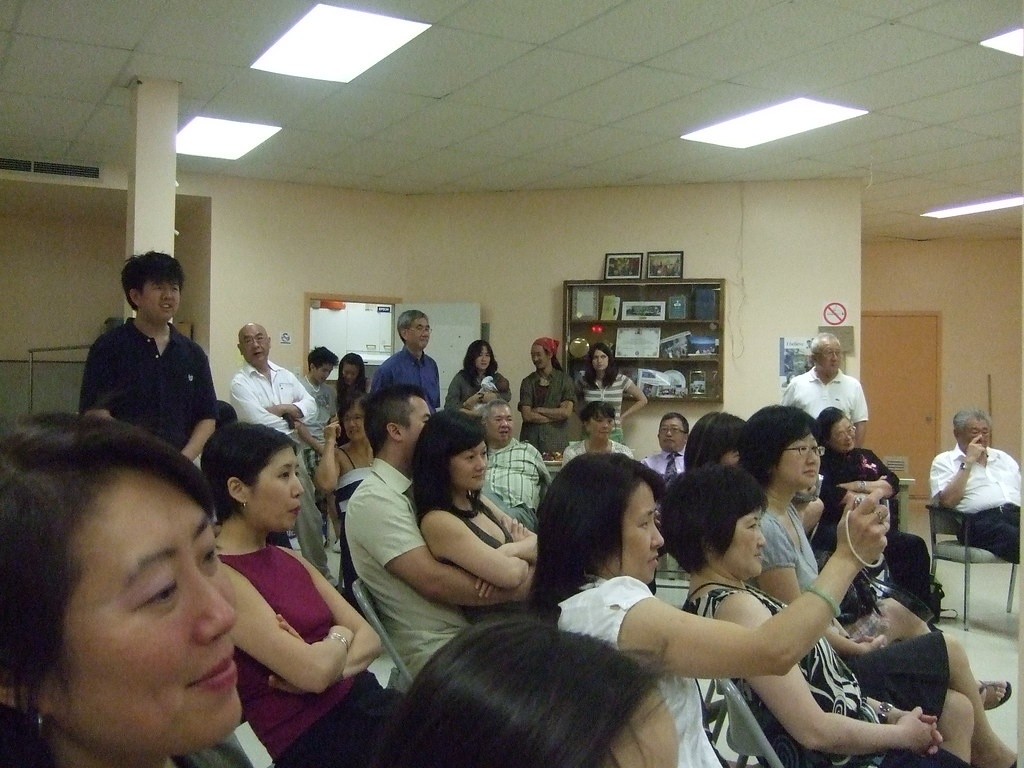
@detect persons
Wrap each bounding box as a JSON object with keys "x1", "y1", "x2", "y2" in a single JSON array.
[
  {"x1": 78, "y1": 252, "x2": 218, "y2": 462},
  {"x1": 298, "y1": 347, "x2": 337, "y2": 547},
  {"x1": 0, "y1": 412, "x2": 251, "y2": 768},
  {"x1": 781, "y1": 333, "x2": 868, "y2": 450},
  {"x1": 336, "y1": 353, "x2": 373, "y2": 399},
  {"x1": 562, "y1": 401, "x2": 633, "y2": 465},
  {"x1": 480, "y1": 398, "x2": 551, "y2": 529},
  {"x1": 517, "y1": 337, "x2": 575, "y2": 453},
  {"x1": 345, "y1": 384, "x2": 536, "y2": 673},
  {"x1": 230, "y1": 323, "x2": 345, "y2": 591},
  {"x1": 575, "y1": 342, "x2": 647, "y2": 444},
  {"x1": 370, "y1": 616, "x2": 678, "y2": 767},
  {"x1": 641, "y1": 412, "x2": 690, "y2": 483},
  {"x1": 444, "y1": 340, "x2": 511, "y2": 416},
  {"x1": 931, "y1": 406, "x2": 1020, "y2": 565},
  {"x1": 531, "y1": 404, "x2": 1017, "y2": 767},
  {"x1": 315, "y1": 398, "x2": 375, "y2": 594},
  {"x1": 200, "y1": 423, "x2": 401, "y2": 767},
  {"x1": 371, "y1": 311, "x2": 440, "y2": 415}
]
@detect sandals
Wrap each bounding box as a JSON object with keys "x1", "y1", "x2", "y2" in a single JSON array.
[{"x1": 978, "y1": 681, "x2": 1013, "y2": 710}]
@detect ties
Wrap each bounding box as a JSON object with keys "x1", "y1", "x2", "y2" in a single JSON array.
[{"x1": 664, "y1": 453, "x2": 677, "y2": 493}]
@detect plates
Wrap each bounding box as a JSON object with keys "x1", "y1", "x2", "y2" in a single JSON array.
[{"x1": 664, "y1": 370, "x2": 686, "y2": 393}]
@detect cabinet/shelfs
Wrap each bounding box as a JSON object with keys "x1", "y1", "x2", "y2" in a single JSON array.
[{"x1": 563, "y1": 279, "x2": 725, "y2": 402}]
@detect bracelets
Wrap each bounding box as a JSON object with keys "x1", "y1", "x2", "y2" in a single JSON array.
[
  {"x1": 878, "y1": 702, "x2": 893, "y2": 723},
  {"x1": 806, "y1": 587, "x2": 841, "y2": 616},
  {"x1": 330, "y1": 634, "x2": 348, "y2": 648}
]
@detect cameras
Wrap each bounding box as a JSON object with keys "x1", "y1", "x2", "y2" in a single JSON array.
[{"x1": 855, "y1": 495, "x2": 889, "y2": 509}]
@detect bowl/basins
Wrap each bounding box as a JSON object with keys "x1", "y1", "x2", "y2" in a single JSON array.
[{"x1": 686, "y1": 336, "x2": 717, "y2": 353}]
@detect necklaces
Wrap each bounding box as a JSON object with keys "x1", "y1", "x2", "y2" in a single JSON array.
[
  {"x1": 458, "y1": 496, "x2": 478, "y2": 517},
  {"x1": 368, "y1": 459, "x2": 372, "y2": 466}
]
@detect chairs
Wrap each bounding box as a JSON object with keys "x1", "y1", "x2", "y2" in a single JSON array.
[
  {"x1": 352, "y1": 579, "x2": 414, "y2": 693},
  {"x1": 926, "y1": 503, "x2": 1018, "y2": 630},
  {"x1": 704, "y1": 676, "x2": 784, "y2": 768}
]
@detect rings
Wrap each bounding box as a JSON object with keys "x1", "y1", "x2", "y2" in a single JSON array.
[{"x1": 874, "y1": 512, "x2": 887, "y2": 530}]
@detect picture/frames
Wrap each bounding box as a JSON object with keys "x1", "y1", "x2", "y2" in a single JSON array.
[
  {"x1": 646, "y1": 251, "x2": 683, "y2": 279},
  {"x1": 604, "y1": 253, "x2": 642, "y2": 278}
]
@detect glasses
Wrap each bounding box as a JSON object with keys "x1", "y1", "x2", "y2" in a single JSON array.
[
  {"x1": 660, "y1": 426, "x2": 688, "y2": 434},
  {"x1": 827, "y1": 425, "x2": 856, "y2": 443},
  {"x1": 788, "y1": 446, "x2": 826, "y2": 456},
  {"x1": 407, "y1": 328, "x2": 432, "y2": 334}
]
